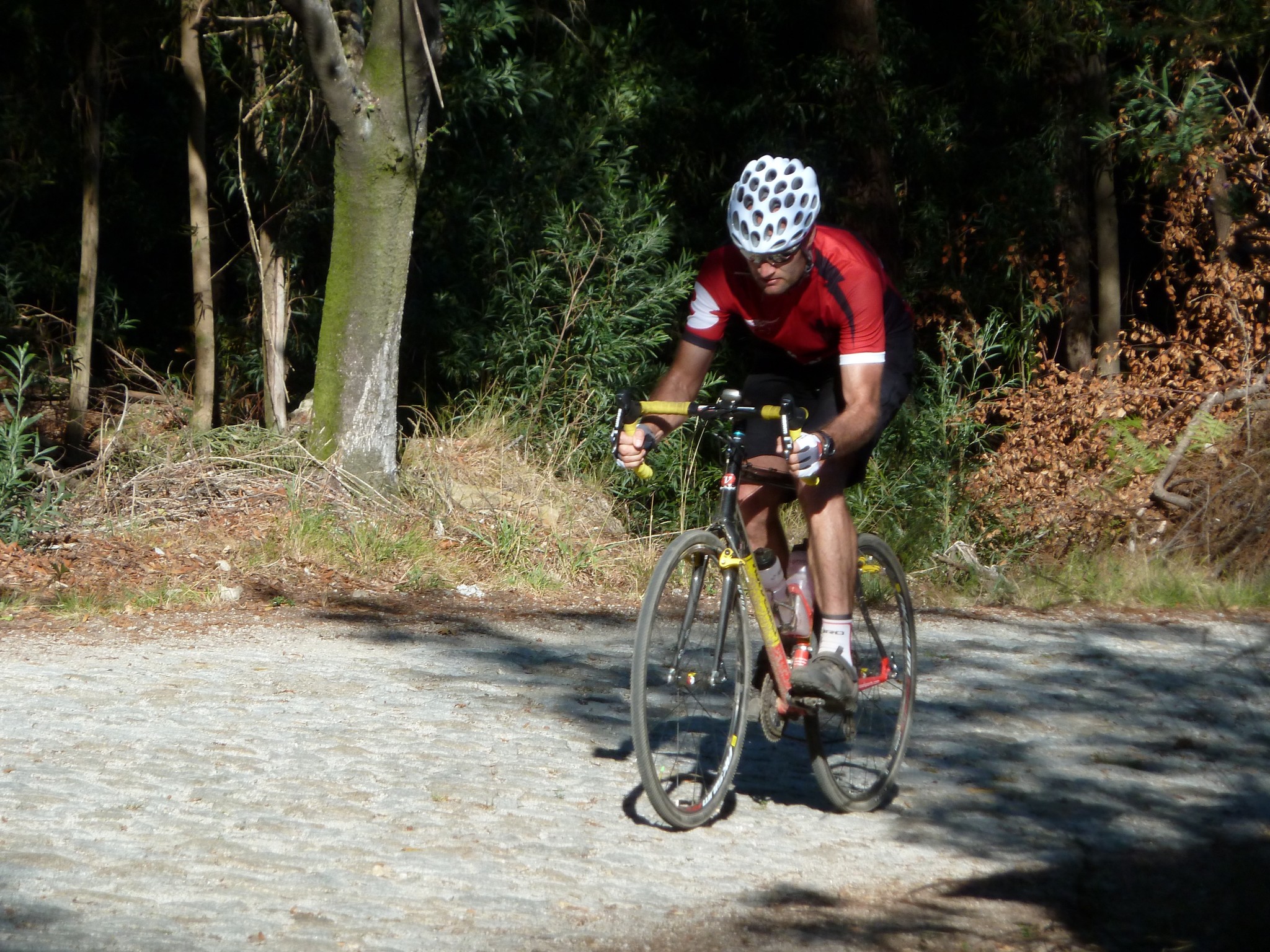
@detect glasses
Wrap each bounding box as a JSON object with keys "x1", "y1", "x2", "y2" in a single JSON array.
[{"x1": 739, "y1": 241, "x2": 803, "y2": 265}]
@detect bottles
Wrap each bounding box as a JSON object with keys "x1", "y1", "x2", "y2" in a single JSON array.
[
  {"x1": 788, "y1": 537, "x2": 814, "y2": 637},
  {"x1": 753, "y1": 547, "x2": 792, "y2": 627}
]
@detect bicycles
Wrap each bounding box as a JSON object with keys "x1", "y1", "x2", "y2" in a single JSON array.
[{"x1": 608, "y1": 389, "x2": 918, "y2": 832}]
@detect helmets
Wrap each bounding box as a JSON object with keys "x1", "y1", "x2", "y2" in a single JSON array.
[{"x1": 726, "y1": 154, "x2": 822, "y2": 254}]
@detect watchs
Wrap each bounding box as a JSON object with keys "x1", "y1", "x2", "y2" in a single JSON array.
[{"x1": 808, "y1": 428, "x2": 836, "y2": 460}]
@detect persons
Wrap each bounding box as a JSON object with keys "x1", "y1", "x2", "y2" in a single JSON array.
[{"x1": 615, "y1": 152, "x2": 928, "y2": 718}]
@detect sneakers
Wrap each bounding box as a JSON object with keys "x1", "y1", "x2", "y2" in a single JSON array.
[
  {"x1": 750, "y1": 637, "x2": 796, "y2": 689},
  {"x1": 789, "y1": 645, "x2": 860, "y2": 712}
]
